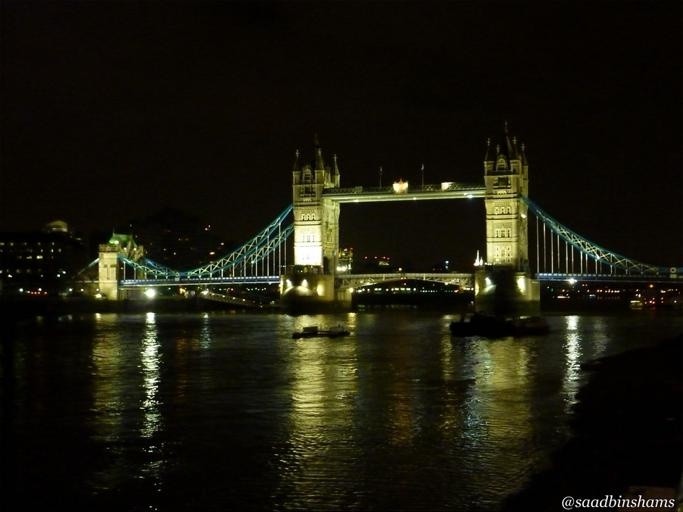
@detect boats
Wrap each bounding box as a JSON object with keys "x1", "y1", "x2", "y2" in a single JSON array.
[
  {"x1": 556, "y1": 293, "x2": 570, "y2": 299},
  {"x1": 448, "y1": 300, "x2": 549, "y2": 335},
  {"x1": 292, "y1": 322, "x2": 348, "y2": 340}
]
